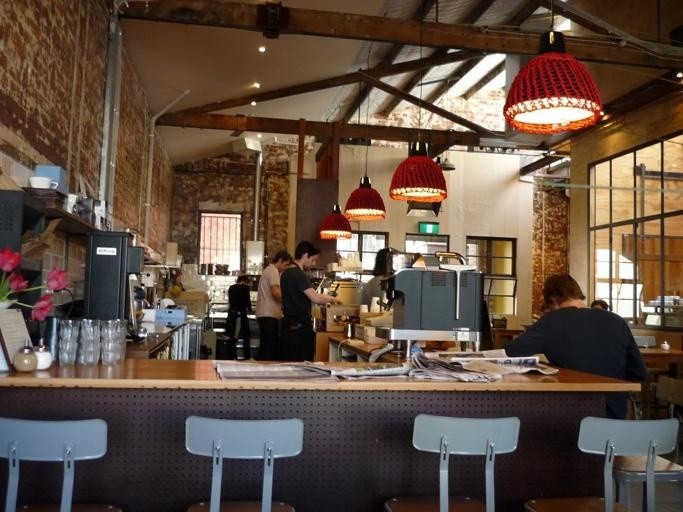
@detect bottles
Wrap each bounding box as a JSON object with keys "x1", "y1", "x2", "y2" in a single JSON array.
[
  {"x1": 12, "y1": 340, "x2": 38, "y2": 372},
  {"x1": 343, "y1": 322, "x2": 353, "y2": 338},
  {"x1": 33, "y1": 338, "x2": 51, "y2": 371},
  {"x1": 306, "y1": 269, "x2": 325, "y2": 278}
]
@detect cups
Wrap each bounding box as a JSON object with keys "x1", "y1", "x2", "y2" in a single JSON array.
[
  {"x1": 358, "y1": 292, "x2": 388, "y2": 323},
  {"x1": 331, "y1": 263, "x2": 338, "y2": 272},
  {"x1": 28, "y1": 176, "x2": 58, "y2": 189},
  {"x1": 200, "y1": 264, "x2": 206, "y2": 275},
  {"x1": 662, "y1": 343, "x2": 670, "y2": 351},
  {"x1": 338, "y1": 251, "x2": 360, "y2": 267},
  {"x1": 56, "y1": 317, "x2": 127, "y2": 366},
  {"x1": 62, "y1": 193, "x2": 79, "y2": 214},
  {"x1": 207, "y1": 264, "x2": 212, "y2": 275}
]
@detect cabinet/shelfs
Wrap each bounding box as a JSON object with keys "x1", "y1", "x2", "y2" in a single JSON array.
[
  {"x1": 124, "y1": 317, "x2": 212, "y2": 363},
  {"x1": 326, "y1": 334, "x2": 405, "y2": 365}
]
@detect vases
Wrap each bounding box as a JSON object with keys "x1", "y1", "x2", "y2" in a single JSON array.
[{"x1": 0, "y1": 299, "x2": 22, "y2": 374}]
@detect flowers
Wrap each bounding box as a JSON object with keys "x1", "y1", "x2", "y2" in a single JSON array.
[{"x1": 0, "y1": 243, "x2": 73, "y2": 323}]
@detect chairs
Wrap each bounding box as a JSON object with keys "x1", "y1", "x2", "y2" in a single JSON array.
[
  {"x1": 181, "y1": 414, "x2": 305, "y2": 511},
  {"x1": 514, "y1": 330, "x2": 683, "y2": 512},
  {"x1": 0, "y1": 412, "x2": 125, "y2": 511},
  {"x1": 376, "y1": 412, "x2": 521, "y2": 510}
]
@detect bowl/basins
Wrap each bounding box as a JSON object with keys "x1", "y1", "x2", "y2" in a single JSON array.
[{"x1": 214, "y1": 264, "x2": 230, "y2": 274}]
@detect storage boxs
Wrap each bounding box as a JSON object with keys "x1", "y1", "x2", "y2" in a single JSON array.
[{"x1": 162, "y1": 287, "x2": 209, "y2": 318}]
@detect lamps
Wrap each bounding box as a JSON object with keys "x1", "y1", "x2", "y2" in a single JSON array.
[
  {"x1": 498, "y1": 0, "x2": 604, "y2": 133},
  {"x1": 339, "y1": 36, "x2": 391, "y2": 221},
  {"x1": 386, "y1": 0, "x2": 449, "y2": 205},
  {"x1": 317, "y1": 107, "x2": 353, "y2": 241},
  {"x1": 436, "y1": 148, "x2": 457, "y2": 172}
]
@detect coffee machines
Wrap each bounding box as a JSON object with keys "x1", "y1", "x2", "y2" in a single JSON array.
[{"x1": 85, "y1": 231, "x2": 148, "y2": 341}]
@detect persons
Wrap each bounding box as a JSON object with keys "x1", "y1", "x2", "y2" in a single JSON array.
[
  {"x1": 505, "y1": 273, "x2": 645, "y2": 419},
  {"x1": 361, "y1": 247, "x2": 394, "y2": 312},
  {"x1": 591, "y1": 300, "x2": 608, "y2": 311},
  {"x1": 256, "y1": 251, "x2": 292, "y2": 360},
  {"x1": 280, "y1": 241, "x2": 341, "y2": 361},
  {"x1": 227, "y1": 276, "x2": 253, "y2": 360}
]
